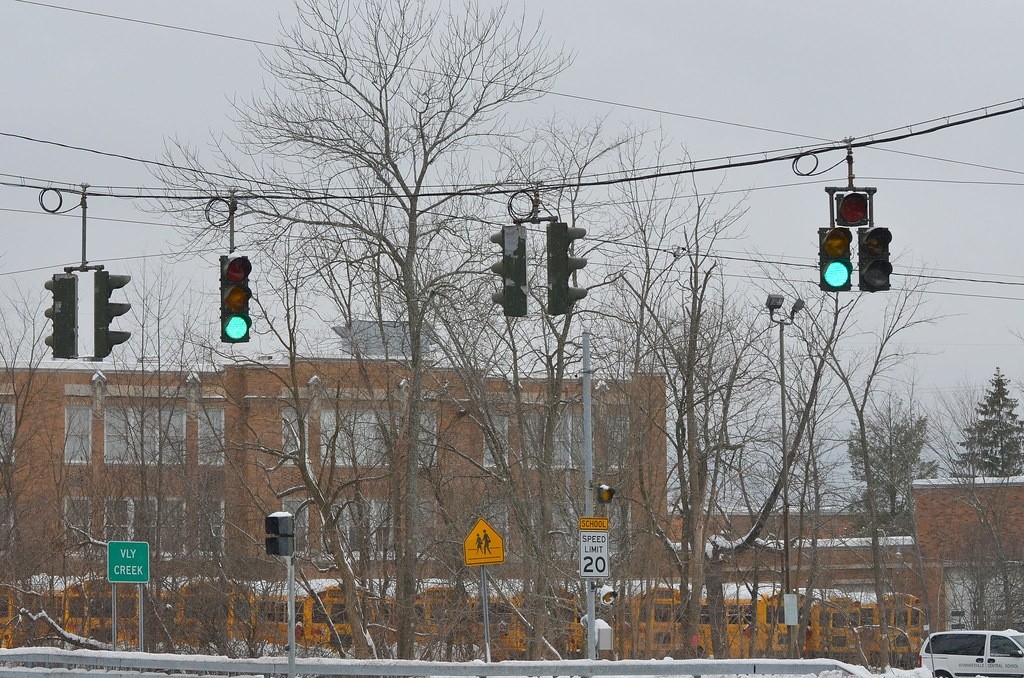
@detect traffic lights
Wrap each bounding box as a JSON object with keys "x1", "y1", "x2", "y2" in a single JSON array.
[
  {"x1": 596, "y1": 484, "x2": 616, "y2": 507},
  {"x1": 488, "y1": 224, "x2": 525, "y2": 317},
  {"x1": 833, "y1": 190, "x2": 870, "y2": 227},
  {"x1": 44, "y1": 274, "x2": 77, "y2": 359},
  {"x1": 548, "y1": 222, "x2": 588, "y2": 315},
  {"x1": 96, "y1": 272, "x2": 132, "y2": 359},
  {"x1": 217, "y1": 252, "x2": 252, "y2": 345},
  {"x1": 859, "y1": 227, "x2": 892, "y2": 290},
  {"x1": 817, "y1": 228, "x2": 853, "y2": 293},
  {"x1": 263, "y1": 512, "x2": 294, "y2": 556}
]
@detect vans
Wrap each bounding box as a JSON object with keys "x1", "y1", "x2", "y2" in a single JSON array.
[{"x1": 918, "y1": 628, "x2": 1024, "y2": 677}]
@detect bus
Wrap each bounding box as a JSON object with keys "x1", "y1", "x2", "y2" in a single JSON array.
[{"x1": 0, "y1": 579, "x2": 925, "y2": 666}]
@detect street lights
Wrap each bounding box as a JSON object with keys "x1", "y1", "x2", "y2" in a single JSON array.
[{"x1": 765, "y1": 293, "x2": 807, "y2": 658}]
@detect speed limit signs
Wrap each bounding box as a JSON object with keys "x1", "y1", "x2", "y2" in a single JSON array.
[{"x1": 579, "y1": 532, "x2": 610, "y2": 579}]
